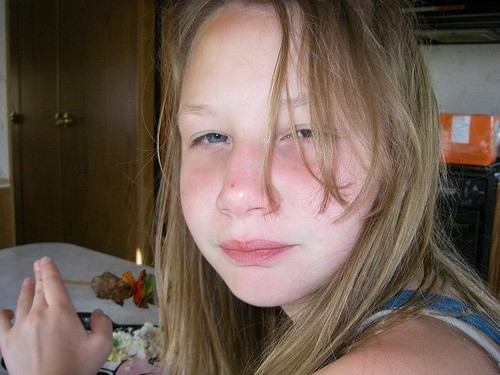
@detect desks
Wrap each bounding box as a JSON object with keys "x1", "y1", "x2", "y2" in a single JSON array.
[{"x1": 0, "y1": 243, "x2": 157, "y2": 375}]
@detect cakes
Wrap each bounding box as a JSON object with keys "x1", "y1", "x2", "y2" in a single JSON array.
[{"x1": 96, "y1": 321, "x2": 163, "y2": 370}]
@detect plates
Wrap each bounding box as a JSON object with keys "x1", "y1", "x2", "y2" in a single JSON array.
[{"x1": 95, "y1": 325, "x2": 162, "y2": 375}]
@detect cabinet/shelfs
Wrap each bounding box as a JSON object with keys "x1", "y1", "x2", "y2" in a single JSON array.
[
  {"x1": 433, "y1": 158, "x2": 500, "y2": 292},
  {"x1": 6, "y1": 0, "x2": 180, "y2": 266}
]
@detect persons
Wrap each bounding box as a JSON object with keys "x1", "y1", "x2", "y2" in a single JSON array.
[{"x1": 0, "y1": 0, "x2": 500, "y2": 375}]
